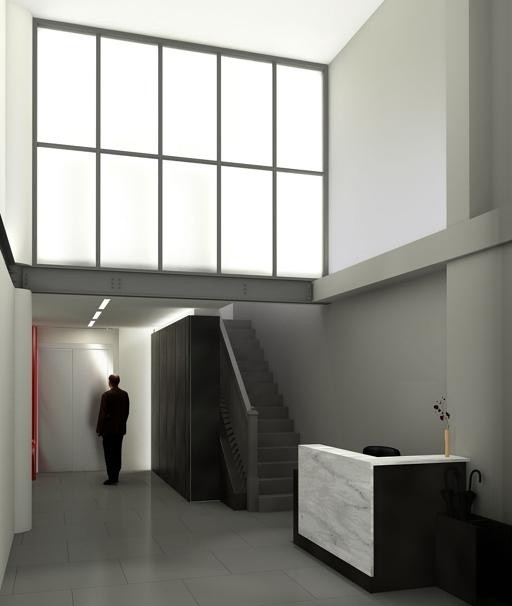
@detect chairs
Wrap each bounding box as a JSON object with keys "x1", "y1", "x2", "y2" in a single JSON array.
[{"x1": 362, "y1": 446, "x2": 401, "y2": 457}]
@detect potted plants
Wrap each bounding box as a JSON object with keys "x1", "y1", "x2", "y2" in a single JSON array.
[{"x1": 433, "y1": 392, "x2": 450, "y2": 457}]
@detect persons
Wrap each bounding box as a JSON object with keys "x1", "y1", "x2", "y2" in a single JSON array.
[{"x1": 95, "y1": 373, "x2": 130, "y2": 485}]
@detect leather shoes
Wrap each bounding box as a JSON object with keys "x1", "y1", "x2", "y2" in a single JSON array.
[{"x1": 104, "y1": 479, "x2": 119, "y2": 485}]
